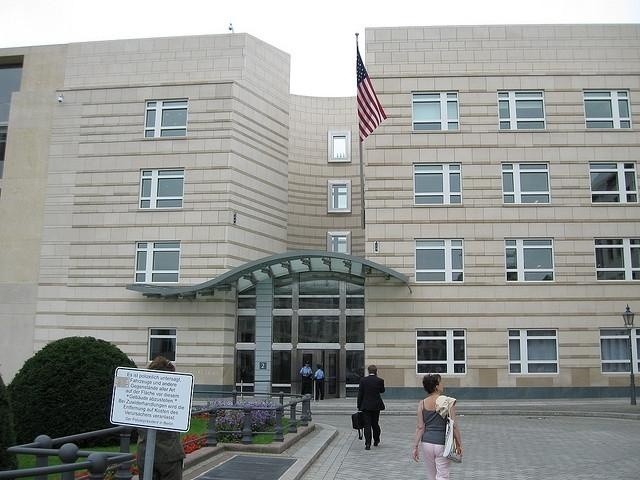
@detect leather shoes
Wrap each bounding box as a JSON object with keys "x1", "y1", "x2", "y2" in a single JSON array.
[
  {"x1": 364, "y1": 442, "x2": 379, "y2": 450},
  {"x1": 315, "y1": 397, "x2": 324, "y2": 401}
]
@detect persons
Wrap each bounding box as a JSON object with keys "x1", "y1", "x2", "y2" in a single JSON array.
[
  {"x1": 313, "y1": 363, "x2": 326, "y2": 401},
  {"x1": 411, "y1": 373, "x2": 462, "y2": 480},
  {"x1": 300, "y1": 359, "x2": 312, "y2": 395},
  {"x1": 356, "y1": 364, "x2": 385, "y2": 450},
  {"x1": 136, "y1": 356, "x2": 188, "y2": 480}
]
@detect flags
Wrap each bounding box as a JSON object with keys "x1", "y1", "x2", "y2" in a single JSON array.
[{"x1": 356, "y1": 42, "x2": 388, "y2": 143}]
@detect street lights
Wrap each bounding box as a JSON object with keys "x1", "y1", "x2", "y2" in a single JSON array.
[{"x1": 622, "y1": 304, "x2": 637, "y2": 405}]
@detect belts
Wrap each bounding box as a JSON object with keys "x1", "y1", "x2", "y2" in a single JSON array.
[{"x1": 316, "y1": 379, "x2": 323, "y2": 380}]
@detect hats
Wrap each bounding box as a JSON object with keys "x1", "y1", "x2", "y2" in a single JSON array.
[
  {"x1": 304, "y1": 360, "x2": 310, "y2": 364},
  {"x1": 316, "y1": 364, "x2": 322, "y2": 368}
]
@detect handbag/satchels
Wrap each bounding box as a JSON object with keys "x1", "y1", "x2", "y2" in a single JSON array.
[
  {"x1": 352, "y1": 411, "x2": 364, "y2": 430},
  {"x1": 442, "y1": 416, "x2": 463, "y2": 464}
]
